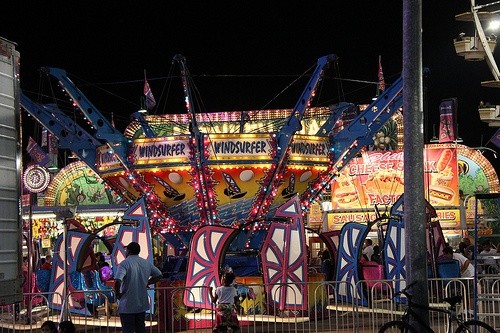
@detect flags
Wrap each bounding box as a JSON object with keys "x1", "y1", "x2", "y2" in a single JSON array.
[
  {"x1": 376, "y1": 58, "x2": 386, "y2": 96},
  {"x1": 26, "y1": 137, "x2": 51, "y2": 166},
  {"x1": 144, "y1": 80, "x2": 156, "y2": 109},
  {"x1": 439, "y1": 99, "x2": 455, "y2": 142}
]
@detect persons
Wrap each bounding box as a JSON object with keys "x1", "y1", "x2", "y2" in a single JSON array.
[
  {"x1": 320, "y1": 239, "x2": 382, "y2": 284},
  {"x1": 443, "y1": 240, "x2": 498, "y2": 277},
  {"x1": 40, "y1": 252, "x2": 109, "y2": 270},
  {"x1": 114, "y1": 242, "x2": 163, "y2": 333},
  {"x1": 208, "y1": 273, "x2": 241, "y2": 333}
]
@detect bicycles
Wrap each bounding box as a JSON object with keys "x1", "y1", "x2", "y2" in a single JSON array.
[{"x1": 377, "y1": 278, "x2": 496, "y2": 333}]
